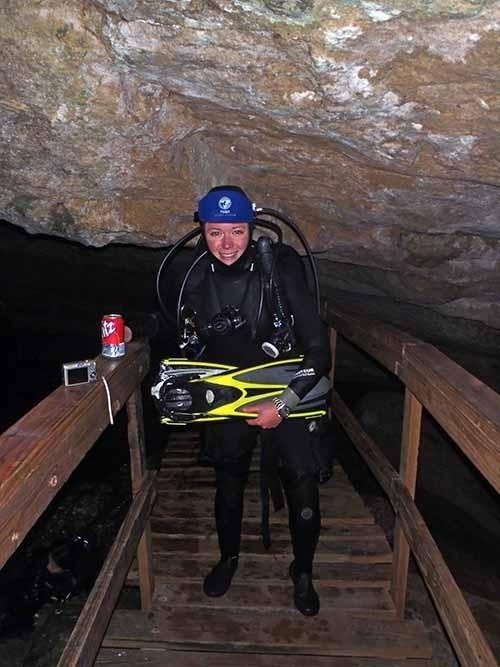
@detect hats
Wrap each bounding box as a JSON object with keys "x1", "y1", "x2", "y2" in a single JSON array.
[{"x1": 198, "y1": 185, "x2": 255, "y2": 224}]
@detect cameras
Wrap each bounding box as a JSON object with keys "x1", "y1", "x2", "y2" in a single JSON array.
[{"x1": 63, "y1": 359, "x2": 98, "y2": 388}]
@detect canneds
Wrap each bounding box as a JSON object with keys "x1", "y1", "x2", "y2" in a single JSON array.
[{"x1": 100, "y1": 314, "x2": 126, "y2": 358}]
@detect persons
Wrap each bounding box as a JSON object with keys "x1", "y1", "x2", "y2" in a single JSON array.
[{"x1": 149, "y1": 185, "x2": 337, "y2": 617}]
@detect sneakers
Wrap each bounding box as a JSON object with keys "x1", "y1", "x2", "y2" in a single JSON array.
[
  {"x1": 203, "y1": 553, "x2": 239, "y2": 598},
  {"x1": 289, "y1": 559, "x2": 321, "y2": 617}
]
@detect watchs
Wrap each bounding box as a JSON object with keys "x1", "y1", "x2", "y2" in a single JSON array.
[{"x1": 274, "y1": 395, "x2": 290, "y2": 419}]
